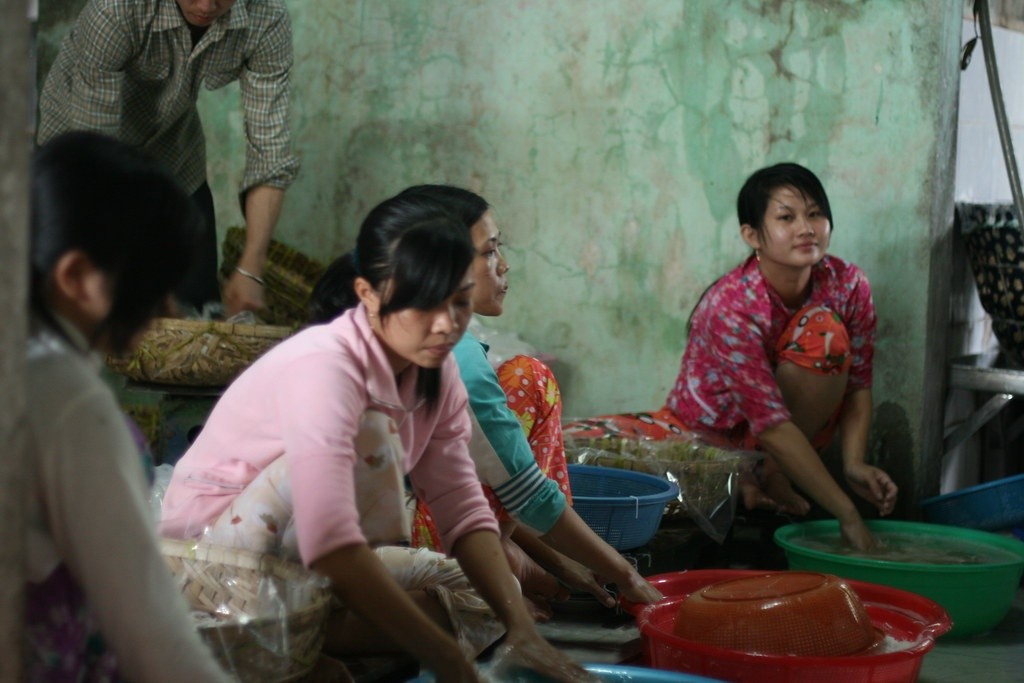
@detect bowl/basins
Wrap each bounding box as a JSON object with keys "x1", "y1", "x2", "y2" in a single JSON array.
[
  {"x1": 917, "y1": 472, "x2": 1024, "y2": 533},
  {"x1": 403, "y1": 662, "x2": 728, "y2": 683},
  {"x1": 618, "y1": 566, "x2": 788, "y2": 615},
  {"x1": 638, "y1": 579, "x2": 952, "y2": 683},
  {"x1": 772, "y1": 517, "x2": 1024, "y2": 641}
]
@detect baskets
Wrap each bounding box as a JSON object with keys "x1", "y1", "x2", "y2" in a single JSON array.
[
  {"x1": 671, "y1": 570, "x2": 887, "y2": 659},
  {"x1": 118, "y1": 317, "x2": 297, "y2": 388},
  {"x1": 560, "y1": 460, "x2": 682, "y2": 552}
]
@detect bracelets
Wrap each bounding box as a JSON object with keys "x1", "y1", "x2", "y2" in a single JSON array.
[{"x1": 237, "y1": 266, "x2": 265, "y2": 284}]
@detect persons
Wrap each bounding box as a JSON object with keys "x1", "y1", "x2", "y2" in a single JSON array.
[
  {"x1": 398, "y1": 184, "x2": 664, "y2": 620},
  {"x1": 37, "y1": 0, "x2": 304, "y2": 318},
  {"x1": 561, "y1": 162, "x2": 898, "y2": 549},
  {"x1": 22, "y1": 132, "x2": 235, "y2": 683},
  {"x1": 157, "y1": 196, "x2": 604, "y2": 683}
]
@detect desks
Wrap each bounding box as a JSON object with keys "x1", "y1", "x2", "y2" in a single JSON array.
[{"x1": 938, "y1": 351, "x2": 1024, "y2": 496}]
